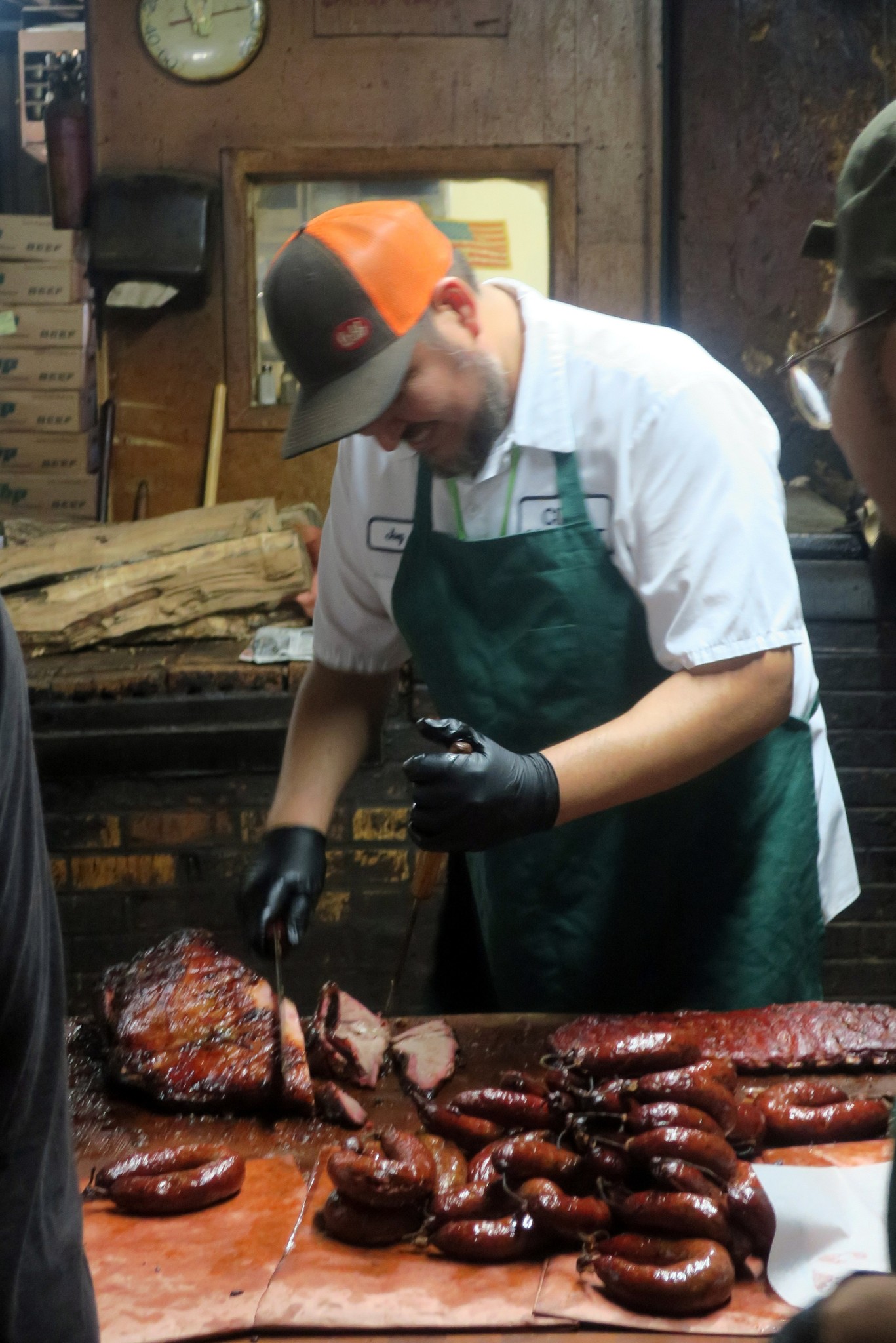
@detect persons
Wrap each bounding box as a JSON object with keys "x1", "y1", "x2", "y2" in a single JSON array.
[
  {"x1": 0, "y1": 591, "x2": 102, "y2": 1343},
  {"x1": 237, "y1": 202, "x2": 863, "y2": 1001},
  {"x1": 778, "y1": 100, "x2": 895, "y2": 1343}
]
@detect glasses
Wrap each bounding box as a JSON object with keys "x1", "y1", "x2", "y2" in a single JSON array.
[{"x1": 771, "y1": 306, "x2": 894, "y2": 432}]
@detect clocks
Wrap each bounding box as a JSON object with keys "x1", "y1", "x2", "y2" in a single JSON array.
[{"x1": 136, "y1": 0, "x2": 268, "y2": 84}]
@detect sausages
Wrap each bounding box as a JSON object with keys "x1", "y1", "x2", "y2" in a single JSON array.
[
  {"x1": 93, "y1": 1141, "x2": 245, "y2": 1213},
  {"x1": 323, "y1": 1031, "x2": 889, "y2": 1311}
]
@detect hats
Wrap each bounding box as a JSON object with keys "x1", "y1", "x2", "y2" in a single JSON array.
[
  {"x1": 264, "y1": 200, "x2": 453, "y2": 462},
  {"x1": 800, "y1": 98, "x2": 896, "y2": 308}
]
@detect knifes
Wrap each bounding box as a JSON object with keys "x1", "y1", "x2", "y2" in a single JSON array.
[
  {"x1": 379, "y1": 736, "x2": 472, "y2": 1024},
  {"x1": 266, "y1": 916, "x2": 290, "y2": 1095}
]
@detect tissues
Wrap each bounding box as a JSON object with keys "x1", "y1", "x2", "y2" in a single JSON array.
[{"x1": 86, "y1": 170, "x2": 222, "y2": 311}]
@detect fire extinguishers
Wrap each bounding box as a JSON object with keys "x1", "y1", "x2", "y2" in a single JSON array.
[{"x1": 44, "y1": 56, "x2": 89, "y2": 229}]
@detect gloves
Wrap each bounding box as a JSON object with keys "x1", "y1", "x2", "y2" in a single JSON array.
[
  {"x1": 401, "y1": 718, "x2": 559, "y2": 853},
  {"x1": 237, "y1": 828, "x2": 324, "y2": 958}
]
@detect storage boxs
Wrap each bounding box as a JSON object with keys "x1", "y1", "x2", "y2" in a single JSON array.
[{"x1": 0, "y1": 214, "x2": 107, "y2": 521}]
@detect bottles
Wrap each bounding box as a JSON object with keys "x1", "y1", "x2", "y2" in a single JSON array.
[{"x1": 43, "y1": 50, "x2": 93, "y2": 233}]
